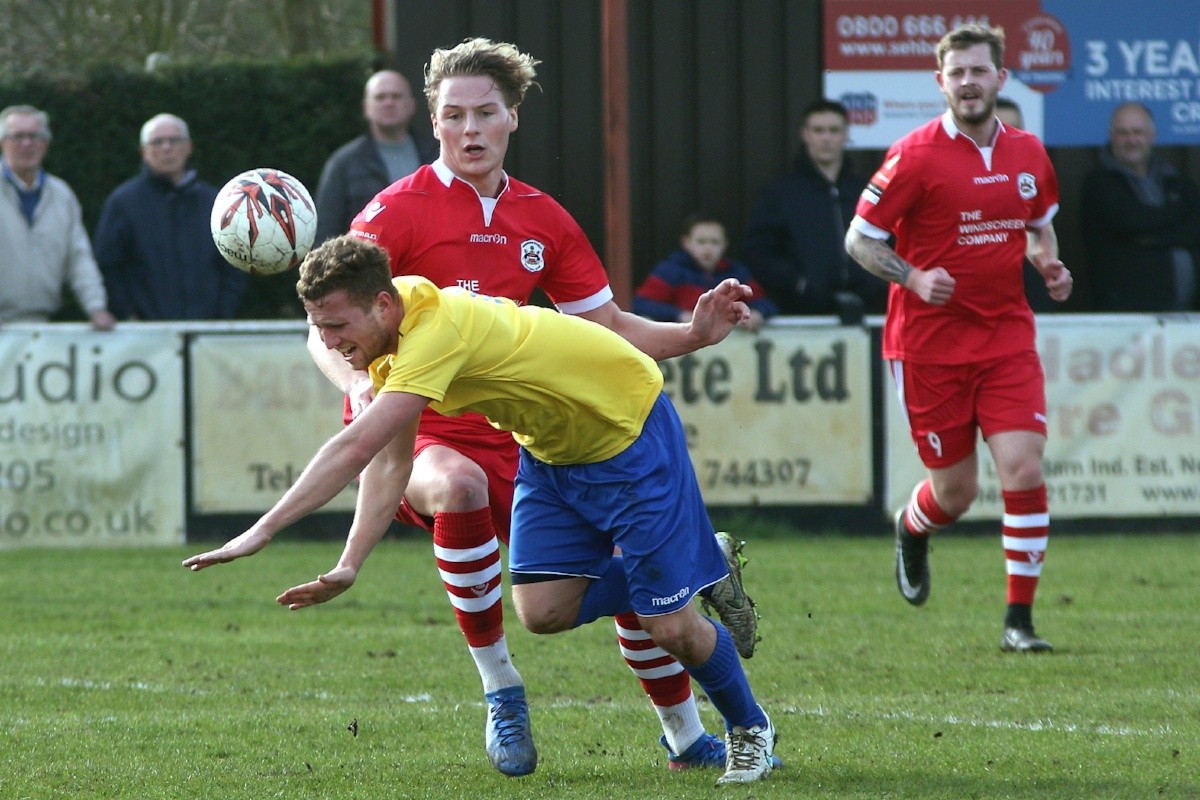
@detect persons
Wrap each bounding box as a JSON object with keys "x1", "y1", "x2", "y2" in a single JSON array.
[
  {"x1": 995, "y1": 100, "x2": 1074, "y2": 314},
  {"x1": 306, "y1": 33, "x2": 784, "y2": 778},
  {"x1": 843, "y1": 25, "x2": 1074, "y2": 653},
  {"x1": 93, "y1": 113, "x2": 250, "y2": 323},
  {"x1": 0, "y1": 104, "x2": 115, "y2": 331},
  {"x1": 1075, "y1": 103, "x2": 1200, "y2": 312},
  {"x1": 310, "y1": 69, "x2": 439, "y2": 253},
  {"x1": 740, "y1": 101, "x2": 897, "y2": 327},
  {"x1": 181, "y1": 235, "x2": 780, "y2": 789},
  {"x1": 631, "y1": 212, "x2": 776, "y2": 331}
]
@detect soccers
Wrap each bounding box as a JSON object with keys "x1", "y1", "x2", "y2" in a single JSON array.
[{"x1": 210, "y1": 168, "x2": 316, "y2": 279}]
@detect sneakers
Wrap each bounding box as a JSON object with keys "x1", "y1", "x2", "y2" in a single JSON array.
[
  {"x1": 998, "y1": 624, "x2": 1051, "y2": 654},
  {"x1": 716, "y1": 703, "x2": 777, "y2": 789},
  {"x1": 658, "y1": 733, "x2": 782, "y2": 771},
  {"x1": 700, "y1": 531, "x2": 762, "y2": 660},
  {"x1": 484, "y1": 686, "x2": 537, "y2": 777},
  {"x1": 895, "y1": 507, "x2": 931, "y2": 606}
]
